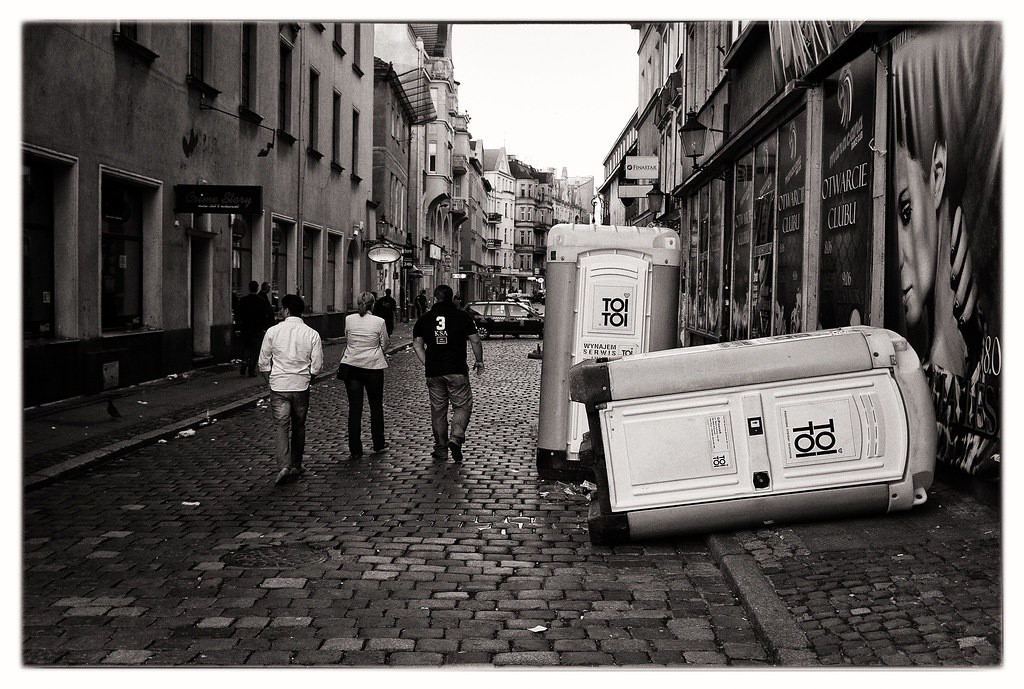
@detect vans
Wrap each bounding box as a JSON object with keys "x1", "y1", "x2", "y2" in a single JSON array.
[{"x1": 463, "y1": 301, "x2": 545, "y2": 338}]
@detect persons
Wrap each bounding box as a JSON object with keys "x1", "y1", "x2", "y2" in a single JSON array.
[
  {"x1": 415, "y1": 290, "x2": 423, "y2": 318},
  {"x1": 259, "y1": 282, "x2": 275, "y2": 334},
  {"x1": 416, "y1": 290, "x2": 427, "y2": 318},
  {"x1": 412, "y1": 284, "x2": 485, "y2": 461},
  {"x1": 233, "y1": 281, "x2": 267, "y2": 377},
  {"x1": 339, "y1": 290, "x2": 390, "y2": 459},
  {"x1": 895, "y1": 74, "x2": 978, "y2": 382},
  {"x1": 452, "y1": 285, "x2": 547, "y2": 310},
  {"x1": 370, "y1": 291, "x2": 382, "y2": 317},
  {"x1": 256, "y1": 293, "x2": 326, "y2": 485},
  {"x1": 379, "y1": 288, "x2": 398, "y2": 338}
]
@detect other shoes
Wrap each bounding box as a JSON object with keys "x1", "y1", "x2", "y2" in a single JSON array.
[
  {"x1": 249, "y1": 372, "x2": 257, "y2": 376},
  {"x1": 291, "y1": 466, "x2": 304, "y2": 473},
  {"x1": 447, "y1": 436, "x2": 463, "y2": 461},
  {"x1": 352, "y1": 450, "x2": 363, "y2": 457},
  {"x1": 274, "y1": 467, "x2": 289, "y2": 483},
  {"x1": 373, "y1": 441, "x2": 389, "y2": 451},
  {"x1": 240, "y1": 363, "x2": 247, "y2": 375},
  {"x1": 432, "y1": 451, "x2": 447, "y2": 459}
]
